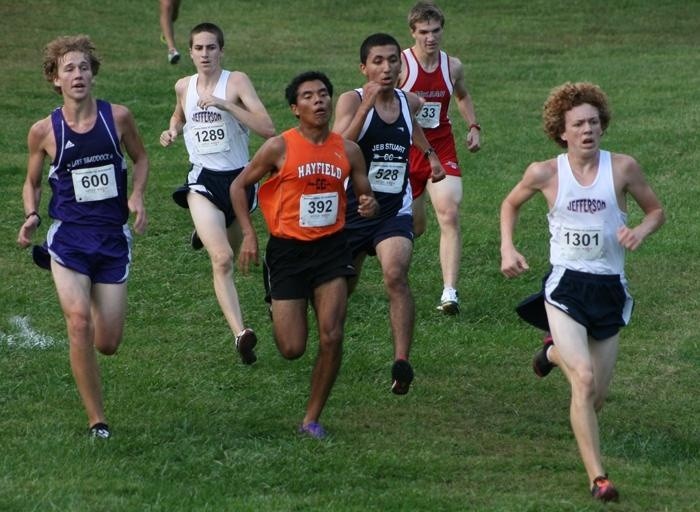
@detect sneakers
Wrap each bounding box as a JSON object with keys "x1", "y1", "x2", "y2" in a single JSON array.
[
  {"x1": 191, "y1": 228, "x2": 203, "y2": 250},
  {"x1": 438, "y1": 285, "x2": 459, "y2": 313},
  {"x1": 90, "y1": 424, "x2": 107, "y2": 438},
  {"x1": 160, "y1": 32, "x2": 167, "y2": 47},
  {"x1": 299, "y1": 421, "x2": 326, "y2": 437},
  {"x1": 391, "y1": 358, "x2": 414, "y2": 395},
  {"x1": 168, "y1": 48, "x2": 180, "y2": 63},
  {"x1": 589, "y1": 473, "x2": 618, "y2": 500},
  {"x1": 236, "y1": 328, "x2": 258, "y2": 365},
  {"x1": 534, "y1": 335, "x2": 556, "y2": 379}
]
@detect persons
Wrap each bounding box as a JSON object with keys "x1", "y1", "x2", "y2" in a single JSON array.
[
  {"x1": 498, "y1": 81, "x2": 665, "y2": 501},
  {"x1": 230, "y1": 72, "x2": 380, "y2": 441},
  {"x1": 158, "y1": 3, "x2": 179, "y2": 59},
  {"x1": 397, "y1": 1, "x2": 482, "y2": 314},
  {"x1": 331, "y1": 32, "x2": 447, "y2": 393},
  {"x1": 158, "y1": 22, "x2": 275, "y2": 365},
  {"x1": 18, "y1": 36, "x2": 147, "y2": 438}
]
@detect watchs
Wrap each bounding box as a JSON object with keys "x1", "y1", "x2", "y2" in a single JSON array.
[
  {"x1": 468, "y1": 123, "x2": 482, "y2": 131},
  {"x1": 26, "y1": 212, "x2": 43, "y2": 228}
]
